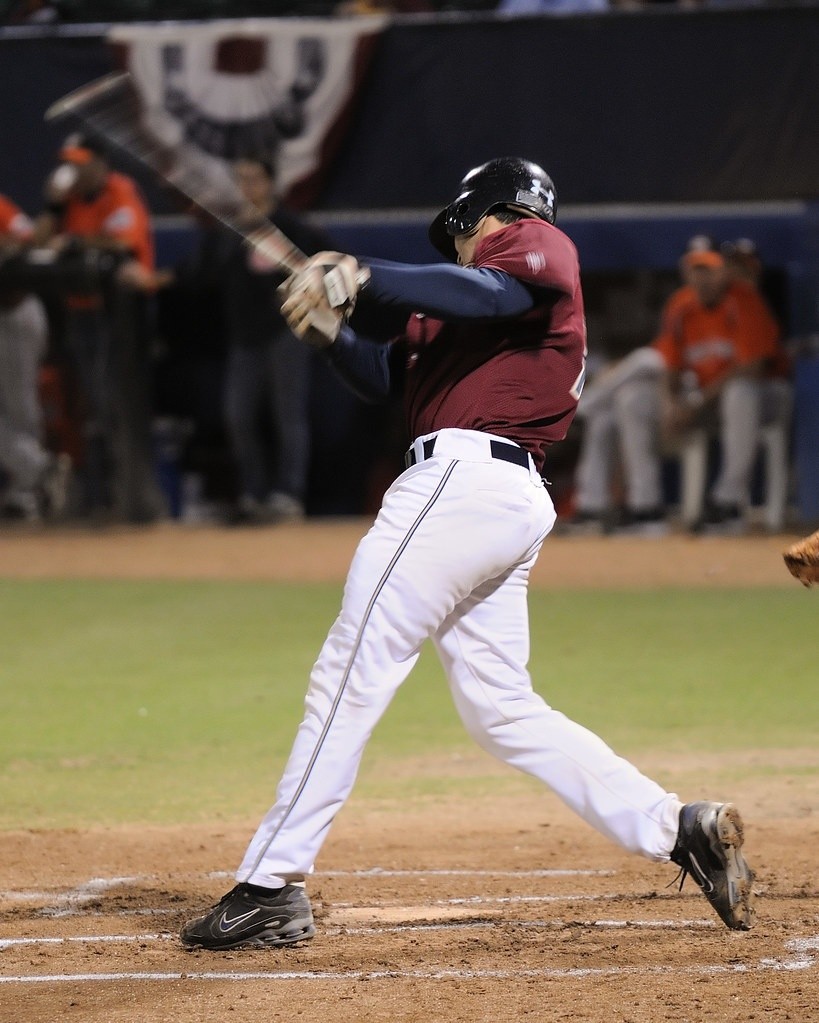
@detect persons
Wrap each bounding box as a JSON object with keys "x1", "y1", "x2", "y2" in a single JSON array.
[
  {"x1": 564, "y1": 234, "x2": 778, "y2": 529},
  {"x1": 174, "y1": 156, "x2": 752, "y2": 946},
  {"x1": 0, "y1": 134, "x2": 332, "y2": 524}
]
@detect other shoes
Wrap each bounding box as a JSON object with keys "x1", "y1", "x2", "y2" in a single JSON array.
[
  {"x1": 602, "y1": 499, "x2": 666, "y2": 534},
  {"x1": 572, "y1": 508, "x2": 610, "y2": 526},
  {"x1": 25, "y1": 494, "x2": 38, "y2": 523},
  {"x1": 689, "y1": 500, "x2": 742, "y2": 535},
  {"x1": 36, "y1": 452, "x2": 70, "y2": 524},
  {"x1": 270, "y1": 492, "x2": 304, "y2": 519},
  {"x1": 241, "y1": 499, "x2": 269, "y2": 523}
]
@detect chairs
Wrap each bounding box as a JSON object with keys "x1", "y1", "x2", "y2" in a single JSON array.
[{"x1": 682, "y1": 375, "x2": 792, "y2": 541}]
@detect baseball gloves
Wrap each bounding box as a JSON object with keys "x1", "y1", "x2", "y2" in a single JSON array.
[{"x1": 780, "y1": 529, "x2": 819, "y2": 589}]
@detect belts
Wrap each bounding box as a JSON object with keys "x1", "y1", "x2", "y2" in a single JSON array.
[{"x1": 404, "y1": 435, "x2": 538, "y2": 472}]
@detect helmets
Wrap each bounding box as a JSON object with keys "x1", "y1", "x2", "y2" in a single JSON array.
[{"x1": 428, "y1": 157, "x2": 558, "y2": 264}]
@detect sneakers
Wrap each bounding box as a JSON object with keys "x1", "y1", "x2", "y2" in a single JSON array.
[
  {"x1": 665, "y1": 801, "x2": 755, "y2": 932},
  {"x1": 179, "y1": 882, "x2": 317, "y2": 951}
]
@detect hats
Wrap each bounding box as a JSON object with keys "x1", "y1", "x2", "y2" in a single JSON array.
[
  {"x1": 59, "y1": 133, "x2": 97, "y2": 162},
  {"x1": 678, "y1": 235, "x2": 725, "y2": 269}
]
